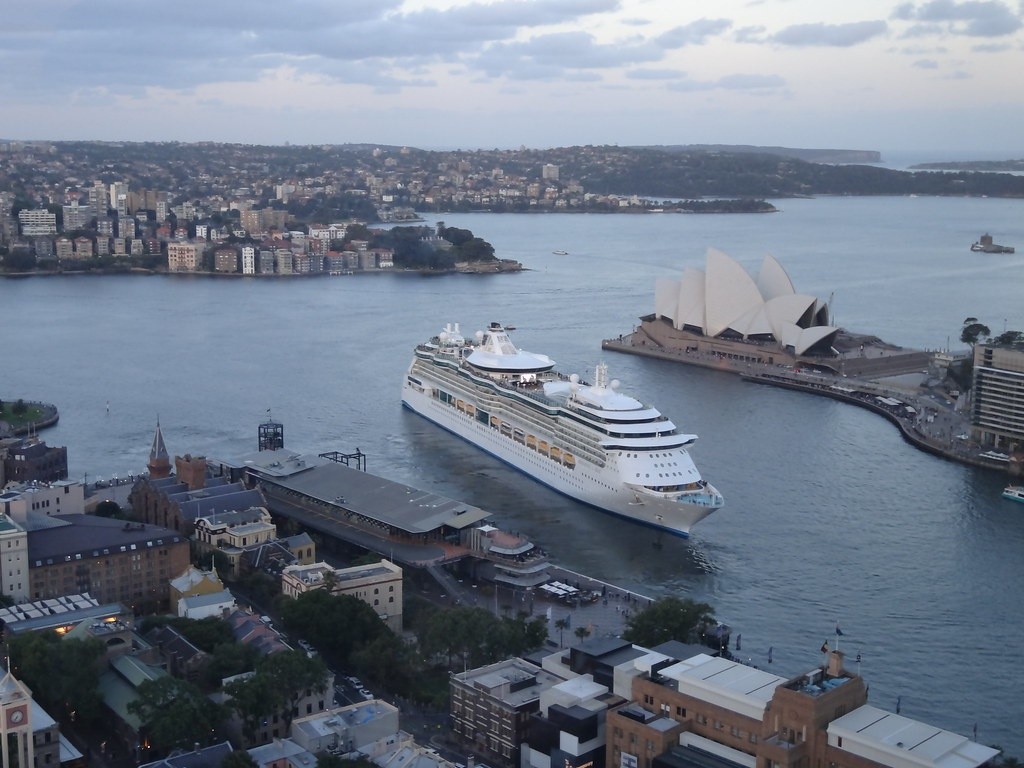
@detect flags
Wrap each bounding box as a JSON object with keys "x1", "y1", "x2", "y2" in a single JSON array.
[
  {"x1": 566, "y1": 612, "x2": 572, "y2": 630},
  {"x1": 587, "y1": 620, "x2": 593, "y2": 635},
  {"x1": 836, "y1": 620, "x2": 843, "y2": 636},
  {"x1": 546, "y1": 606, "x2": 552, "y2": 624}
]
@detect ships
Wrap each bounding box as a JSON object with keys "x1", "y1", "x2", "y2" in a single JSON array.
[{"x1": 400, "y1": 322, "x2": 726, "y2": 539}]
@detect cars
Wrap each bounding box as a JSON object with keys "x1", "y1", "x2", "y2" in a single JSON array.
[
  {"x1": 348, "y1": 676, "x2": 364, "y2": 690},
  {"x1": 309, "y1": 647, "x2": 318, "y2": 657},
  {"x1": 298, "y1": 639, "x2": 310, "y2": 651},
  {"x1": 261, "y1": 615, "x2": 273, "y2": 628},
  {"x1": 359, "y1": 688, "x2": 374, "y2": 700}
]
